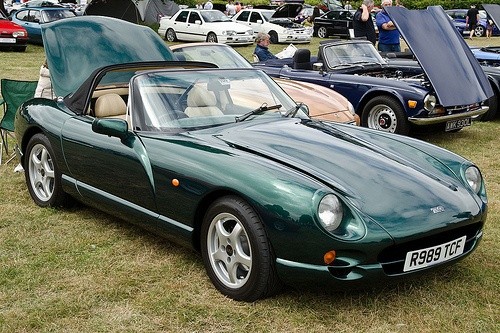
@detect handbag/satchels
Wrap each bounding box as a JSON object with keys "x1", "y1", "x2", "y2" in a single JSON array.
[{"x1": 489, "y1": 19, "x2": 493, "y2": 25}]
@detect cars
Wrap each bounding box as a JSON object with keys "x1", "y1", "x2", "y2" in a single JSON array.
[
  {"x1": 226, "y1": 3, "x2": 312, "y2": 45},
  {"x1": 0, "y1": 0, "x2": 95, "y2": 52},
  {"x1": 157, "y1": 8, "x2": 256, "y2": 48},
  {"x1": 313, "y1": 9, "x2": 378, "y2": 41},
  {"x1": 300, "y1": 0, "x2": 352, "y2": 24}
]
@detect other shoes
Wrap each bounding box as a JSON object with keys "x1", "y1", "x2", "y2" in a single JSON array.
[{"x1": 470, "y1": 37, "x2": 473, "y2": 40}]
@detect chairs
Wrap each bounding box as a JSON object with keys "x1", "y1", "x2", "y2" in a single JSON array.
[
  {"x1": 185, "y1": 89, "x2": 224, "y2": 118},
  {"x1": 95, "y1": 93, "x2": 129, "y2": 122},
  {"x1": 293, "y1": 48, "x2": 312, "y2": 70},
  {"x1": 0, "y1": 78, "x2": 39, "y2": 164}
]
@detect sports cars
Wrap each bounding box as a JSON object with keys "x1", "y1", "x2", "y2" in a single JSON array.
[
  {"x1": 163, "y1": 42, "x2": 361, "y2": 127},
  {"x1": 14, "y1": 15, "x2": 489, "y2": 304},
  {"x1": 379, "y1": 4, "x2": 500, "y2": 123},
  {"x1": 248, "y1": 6, "x2": 494, "y2": 137}
]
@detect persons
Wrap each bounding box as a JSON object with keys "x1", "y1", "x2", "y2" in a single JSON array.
[
  {"x1": 254, "y1": 33, "x2": 279, "y2": 62},
  {"x1": 344, "y1": 0, "x2": 377, "y2": 47},
  {"x1": 376, "y1": 0, "x2": 405, "y2": 53},
  {"x1": 465, "y1": 4, "x2": 494, "y2": 40},
  {"x1": 189, "y1": 0, "x2": 242, "y2": 17}
]
